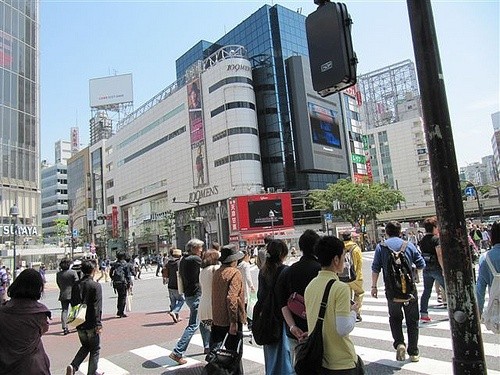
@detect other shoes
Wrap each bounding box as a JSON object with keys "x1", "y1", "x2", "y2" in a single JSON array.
[
  {"x1": 169, "y1": 352, "x2": 188, "y2": 365},
  {"x1": 90, "y1": 372, "x2": 105, "y2": 375},
  {"x1": 169, "y1": 311, "x2": 182, "y2": 323},
  {"x1": 204, "y1": 348, "x2": 209, "y2": 354},
  {"x1": 66, "y1": 364, "x2": 76, "y2": 375},
  {"x1": 410, "y1": 350, "x2": 421, "y2": 362},
  {"x1": 117, "y1": 312, "x2": 128, "y2": 318},
  {"x1": 419, "y1": 316, "x2": 431, "y2": 324},
  {"x1": 395, "y1": 344, "x2": 407, "y2": 362},
  {"x1": 64, "y1": 330, "x2": 70, "y2": 335}
]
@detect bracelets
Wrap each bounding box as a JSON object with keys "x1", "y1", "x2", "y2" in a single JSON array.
[
  {"x1": 290, "y1": 325, "x2": 294, "y2": 331},
  {"x1": 372, "y1": 286, "x2": 377, "y2": 288}
]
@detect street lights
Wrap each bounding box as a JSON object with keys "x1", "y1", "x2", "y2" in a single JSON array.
[
  {"x1": 9, "y1": 203, "x2": 20, "y2": 279},
  {"x1": 269, "y1": 210, "x2": 275, "y2": 240}
]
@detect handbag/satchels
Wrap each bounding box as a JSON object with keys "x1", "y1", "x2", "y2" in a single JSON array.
[
  {"x1": 292, "y1": 318, "x2": 325, "y2": 375},
  {"x1": 65, "y1": 302, "x2": 87, "y2": 328},
  {"x1": 287, "y1": 292, "x2": 309, "y2": 319},
  {"x1": 251, "y1": 291, "x2": 282, "y2": 346}
]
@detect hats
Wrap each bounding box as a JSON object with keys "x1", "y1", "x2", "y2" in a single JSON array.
[{"x1": 216, "y1": 244, "x2": 245, "y2": 264}]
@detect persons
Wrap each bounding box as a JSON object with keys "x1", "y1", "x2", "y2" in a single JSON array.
[
  {"x1": 433, "y1": 216, "x2": 493, "y2": 307},
  {"x1": 258, "y1": 238, "x2": 296, "y2": 375},
  {"x1": 196, "y1": 146, "x2": 204, "y2": 184},
  {"x1": 65, "y1": 259, "x2": 104, "y2": 375},
  {"x1": 419, "y1": 217, "x2": 445, "y2": 322},
  {"x1": 134, "y1": 253, "x2": 168, "y2": 284},
  {"x1": 246, "y1": 242, "x2": 295, "y2": 264},
  {"x1": 188, "y1": 86, "x2": 201, "y2": 120},
  {"x1": 304, "y1": 236, "x2": 368, "y2": 375},
  {"x1": 336, "y1": 231, "x2": 364, "y2": 322},
  {"x1": 276, "y1": 230, "x2": 322, "y2": 361},
  {"x1": 0, "y1": 265, "x2": 47, "y2": 296},
  {"x1": 0, "y1": 268, "x2": 52, "y2": 375},
  {"x1": 476, "y1": 221, "x2": 500, "y2": 318},
  {"x1": 381, "y1": 230, "x2": 424, "y2": 248},
  {"x1": 371, "y1": 221, "x2": 426, "y2": 361},
  {"x1": 56, "y1": 251, "x2": 136, "y2": 334},
  {"x1": 165, "y1": 237, "x2": 273, "y2": 375}
]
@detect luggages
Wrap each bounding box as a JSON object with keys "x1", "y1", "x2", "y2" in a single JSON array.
[{"x1": 200, "y1": 331, "x2": 244, "y2": 375}]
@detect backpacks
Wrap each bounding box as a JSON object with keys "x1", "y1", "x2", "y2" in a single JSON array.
[
  {"x1": 334, "y1": 243, "x2": 358, "y2": 283},
  {"x1": 481, "y1": 253, "x2": 500, "y2": 336},
  {"x1": 113, "y1": 266, "x2": 126, "y2": 282},
  {"x1": 379, "y1": 241, "x2": 416, "y2": 302}
]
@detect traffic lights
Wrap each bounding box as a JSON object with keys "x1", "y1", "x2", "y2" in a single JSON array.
[{"x1": 97, "y1": 215, "x2": 110, "y2": 220}]
